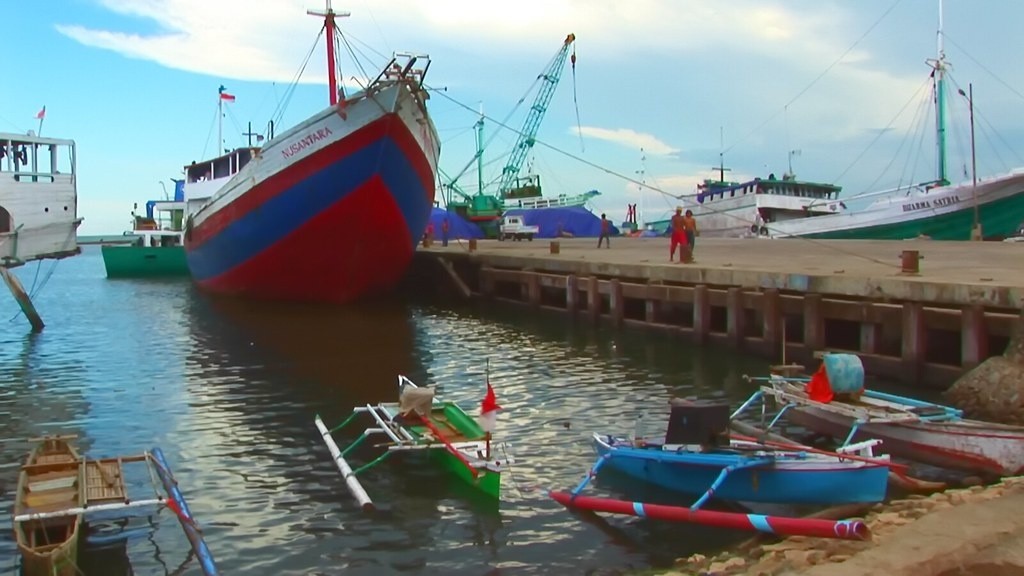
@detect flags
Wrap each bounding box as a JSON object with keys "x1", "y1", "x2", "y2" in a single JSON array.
[
  {"x1": 33, "y1": 108, "x2": 46, "y2": 119},
  {"x1": 220, "y1": 93, "x2": 235, "y2": 100}
]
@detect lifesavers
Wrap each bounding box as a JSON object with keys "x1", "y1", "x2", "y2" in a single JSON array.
[
  {"x1": 760, "y1": 226, "x2": 769, "y2": 235},
  {"x1": 750, "y1": 224, "x2": 758, "y2": 232}
]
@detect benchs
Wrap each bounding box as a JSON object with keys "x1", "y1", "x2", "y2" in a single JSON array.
[
  {"x1": 24, "y1": 486, "x2": 77, "y2": 508},
  {"x1": 27, "y1": 470, "x2": 78, "y2": 491}
]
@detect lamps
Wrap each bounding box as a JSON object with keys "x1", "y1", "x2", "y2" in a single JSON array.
[{"x1": 257, "y1": 135, "x2": 264, "y2": 141}]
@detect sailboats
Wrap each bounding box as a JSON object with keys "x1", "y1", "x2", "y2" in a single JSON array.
[{"x1": 645, "y1": 1, "x2": 1024, "y2": 241}]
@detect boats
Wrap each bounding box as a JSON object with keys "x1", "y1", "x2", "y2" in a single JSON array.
[
  {"x1": 419, "y1": 174, "x2": 622, "y2": 239},
  {"x1": 730, "y1": 317, "x2": 1023, "y2": 494},
  {"x1": 314, "y1": 358, "x2": 516, "y2": 509},
  {"x1": 102, "y1": 178, "x2": 191, "y2": 279},
  {"x1": 0, "y1": 433, "x2": 219, "y2": 576},
  {"x1": 183, "y1": 0, "x2": 443, "y2": 309},
  {"x1": 547, "y1": 403, "x2": 912, "y2": 539}
]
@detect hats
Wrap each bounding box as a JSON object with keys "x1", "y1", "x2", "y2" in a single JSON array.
[{"x1": 676, "y1": 206, "x2": 682, "y2": 211}]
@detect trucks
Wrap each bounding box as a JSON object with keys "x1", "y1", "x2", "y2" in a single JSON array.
[{"x1": 499, "y1": 215, "x2": 540, "y2": 241}]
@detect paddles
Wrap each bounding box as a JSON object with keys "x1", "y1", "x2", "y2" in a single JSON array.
[{"x1": 730, "y1": 433, "x2": 907, "y2": 478}]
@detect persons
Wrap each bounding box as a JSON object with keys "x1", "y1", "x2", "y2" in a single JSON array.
[
  {"x1": 423, "y1": 221, "x2": 434, "y2": 245},
  {"x1": 597, "y1": 213, "x2": 610, "y2": 249},
  {"x1": 441, "y1": 218, "x2": 449, "y2": 247},
  {"x1": 670, "y1": 205, "x2": 697, "y2": 262}
]
